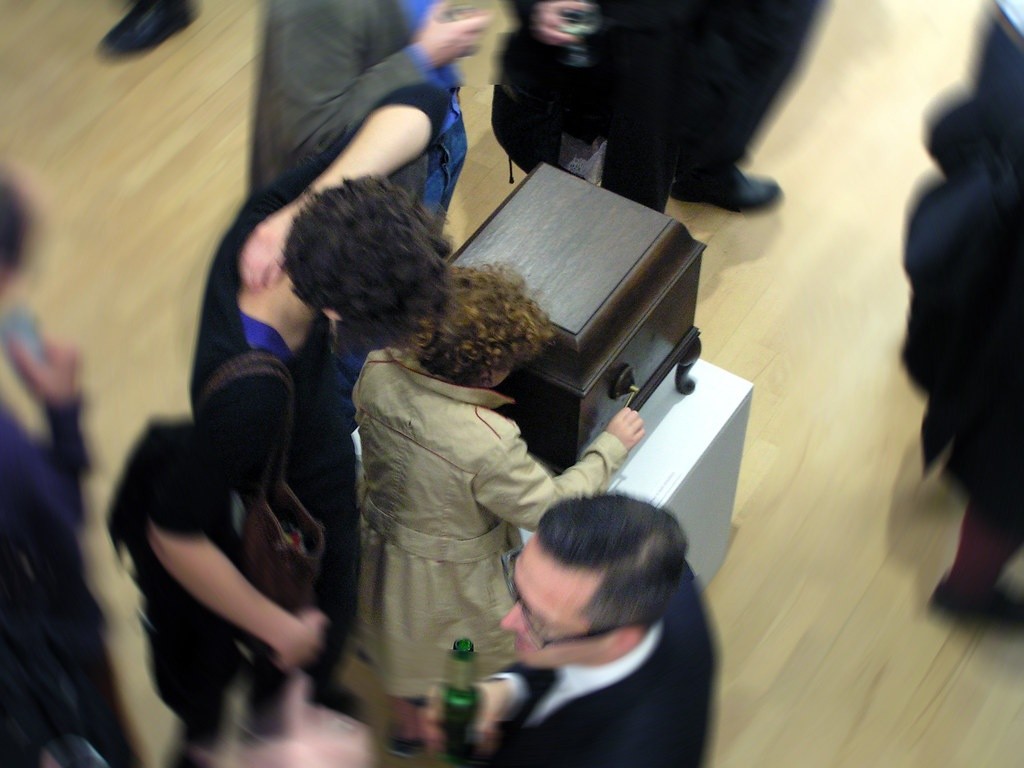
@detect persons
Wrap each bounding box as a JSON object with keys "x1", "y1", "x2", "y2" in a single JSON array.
[
  {"x1": 902, "y1": 97, "x2": 1024, "y2": 625},
  {"x1": 104, "y1": 80, "x2": 470, "y2": 767},
  {"x1": 415, "y1": 491, "x2": 716, "y2": 768},
  {"x1": 340, "y1": 257, "x2": 647, "y2": 759},
  {"x1": 668, "y1": 1, "x2": 831, "y2": 212},
  {"x1": 505, "y1": 0, "x2": 692, "y2": 217},
  {"x1": 252, "y1": 0, "x2": 491, "y2": 277},
  {"x1": 0, "y1": 442, "x2": 139, "y2": 764},
  {"x1": 0, "y1": 168, "x2": 109, "y2": 633},
  {"x1": 97, "y1": 0, "x2": 194, "y2": 58}
]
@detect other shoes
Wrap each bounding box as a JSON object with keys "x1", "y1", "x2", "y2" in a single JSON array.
[
  {"x1": 310, "y1": 678, "x2": 367, "y2": 717},
  {"x1": 383, "y1": 727, "x2": 423, "y2": 751}
]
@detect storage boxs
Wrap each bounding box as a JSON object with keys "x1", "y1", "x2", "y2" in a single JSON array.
[{"x1": 444, "y1": 162, "x2": 701, "y2": 474}]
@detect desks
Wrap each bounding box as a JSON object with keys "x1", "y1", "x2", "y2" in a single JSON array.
[{"x1": 586, "y1": 358, "x2": 753, "y2": 591}]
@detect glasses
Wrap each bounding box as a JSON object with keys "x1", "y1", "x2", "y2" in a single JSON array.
[{"x1": 499, "y1": 549, "x2": 623, "y2": 646}]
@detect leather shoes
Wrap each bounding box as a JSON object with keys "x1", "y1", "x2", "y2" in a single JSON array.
[
  {"x1": 95, "y1": 1, "x2": 191, "y2": 54},
  {"x1": 929, "y1": 574, "x2": 1024, "y2": 634},
  {"x1": 672, "y1": 158, "x2": 780, "y2": 214}
]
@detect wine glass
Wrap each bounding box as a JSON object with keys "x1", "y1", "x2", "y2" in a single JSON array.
[
  {"x1": 561, "y1": 1, "x2": 599, "y2": 66},
  {"x1": 449, "y1": 5, "x2": 477, "y2": 59}
]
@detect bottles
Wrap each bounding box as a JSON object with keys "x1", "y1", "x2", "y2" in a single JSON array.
[{"x1": 439, "y1": 637, "x2": 481, "y2": 768}]
